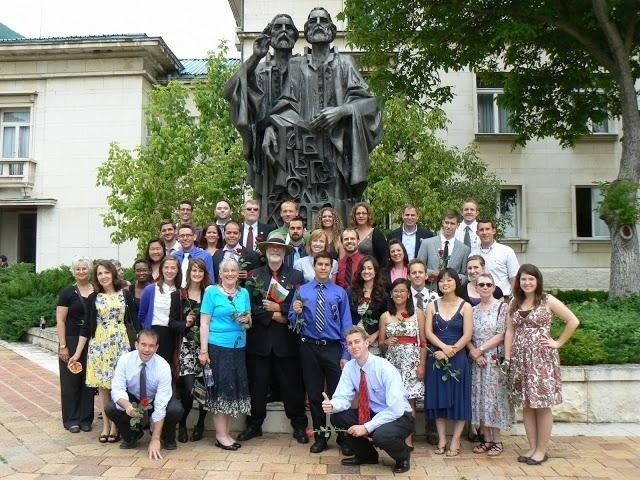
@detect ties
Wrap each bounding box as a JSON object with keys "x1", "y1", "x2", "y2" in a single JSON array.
[
  {"x1": 292, "y1": 246, "x2": 300, "y2": 265},
  {"x1": 181, "y1": 252, "x2": 190, "y2": 289},
  {"x1": 246, "y1": 225, "x2": 253, "y2": 251},
  {"x1": 140, "y1": 362, "x2": 149, "y2": 427},
  {"x1": 464, "y1": 226, "x2": 471, "y2": 253},
  {"x1": 444, "y1": 240, "x2": 449, "y2": 268},
  {"x1": 345, "y1": 257, "x2": 352, "y2": 286},
  {"x1": 167, "y1": 249, "x2": 172, "y2": 255},
  {"x1": 415, "y1": 292, "x2": 423, "y2": 312},
  {"x1": 219, "y1": 225, "x2": 225, "y2": 241},
  {"x1": 357, "y1": 369, "x2": 371, "y2": 439},
  {"x1": 316, "y1": 283, "x2": 325, "y2": 333},
  {"x1": 272, "y1": 271, "x2": 278, "y2": 282}
]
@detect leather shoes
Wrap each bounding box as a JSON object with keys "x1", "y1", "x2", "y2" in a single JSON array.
[
  {"x1": 120, "y1": 431, "x2": 144, "y2": 449},
  {"x1": 342, "y1": 455, "x2": 379, "y2": 465},
  {"x1": 214, "y1": 437, "x2": 240, "y2": 451},
  {"x1": 237, "y1": 427, "x2": 263, "y2": 441},
  {"x1": 527, "y1": 454, "x2": 548, "y2": 464},
  {"x1": 469, "y1": 428, "x2": 479, "y2": 442},
  {"x1": 82, "y1": 426, "x2": 92, "y2": 432},
  {"x1": 404, "y1": 439, "x2": 414, "y2": 451},
  {"x1": 311, "y1": 440, "x2": 327, "y2": 453},
  {"x1": 518, "y1": 455, "x2": 530, "y2": 463},
  {"x1": 342, "y1": 445, "x2": 354, "y2": 456},
  {"x1": 395, "y1": 457, "x2": 410, "y2": 473},
  {"x1": 293, "y1": 430, "x2": 309, "y2": 443},
  {"x1": 163, "y1": 440, "x2": 176, "y2": 449},
  {"x1": 477, "y1": 428, "x2": 486, "y2": 443},
  {"x1": 69, "y1": 425, "x2": 80, "y2": 433}
]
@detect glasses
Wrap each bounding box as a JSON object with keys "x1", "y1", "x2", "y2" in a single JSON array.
[
  {"x1": 179, "y1": 234, "x2": 192, "y2": 237},
  {"x1": 331, "y1": 304, "x2": 339, "y2": 322},
  {"x1": 355, "y1": 212, "x2": 368, "y2": 215},
  {"x1": 179, "y1": 208, "x2": 191, "y2": 211},
  {"x1": 477, "y1": 283, "x2": 493, "y2": 287},
  {"x1": 244, "y1": 207, "x2": 258, "y2": 211}
]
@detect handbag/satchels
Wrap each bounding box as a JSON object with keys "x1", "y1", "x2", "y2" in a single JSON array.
[
  {"x1": 172, "y1": 350, "x2": 180, "y2": 382},
  {"x1": 126, "y1": 321, "x2": 136, "y2": 351},
  {"x1": 496, "y1": 357, "x2": 505, "y2": 366},
  {"x1": 190, "y1": 378, "x2": 211, "y2": 407}
]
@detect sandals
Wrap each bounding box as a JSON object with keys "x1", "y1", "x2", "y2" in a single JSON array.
[
  {"x1": 487, "y1": 442, "x2": 503, "y2": 456},
  {"x1": 473, "y1": 441, "x2": 493, "y2": 454}
]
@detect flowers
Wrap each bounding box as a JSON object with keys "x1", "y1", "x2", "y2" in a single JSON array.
[
  {"x1": 359, "y1": 246, "x2": 453, "y2": 340},
  {"x1": 178, "y1": 235, "x2": 307, "y2": 348},
  {"x1": 418, "y1": 340, "x2": 461, "y2": 386},
  {"x1": 303, "y1": 424, "x2": 375, "y2": 445},
  {"x1": 490, "y1": 353, "x2": 524, "y2": 412},
  {"x1": 129, "y1": 395, "x2": 152, "y2": 435}
]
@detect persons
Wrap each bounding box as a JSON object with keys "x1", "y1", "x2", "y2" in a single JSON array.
[
  {"x1": 0, "y1": 254, "x2": 9, "y2": 268},
  {"x1": 220, "y1": 14, "x2": 303, "y2": 231},
  {"x1": 54, "y1": 197, "x2": 580, "y2": 473},
  {"x1": 261, "y1": 6, "x2": 383, "y2": 226}
]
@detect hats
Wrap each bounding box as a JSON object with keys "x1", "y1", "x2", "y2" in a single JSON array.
[{"x1": 258, "y1": 232, "x2": 293, "y2": 256}]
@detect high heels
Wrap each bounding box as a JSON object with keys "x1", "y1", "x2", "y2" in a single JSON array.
[
  {"x1": 99, "y1": 430, "x2": 110, "y2": 442},
  {"x1": 178, "y1": 428, "x2": 188, "y2": 443},
  {"x1": 446, "y1": 443, "x2": 462, "y2": 456},
  {"x1": 108, "y1": 429, "x2": 120, "y2": 442},
  {"x1": 434, "y1": 440, "x2": 448, "y2": 456},
  {"x1": 190, "y1": 425, "x2": 204, "y2": 441}
]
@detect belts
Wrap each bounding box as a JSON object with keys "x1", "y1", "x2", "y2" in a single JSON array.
[{"x1": 304, "y1": 338, "x2": 341, "y2": 345}]
